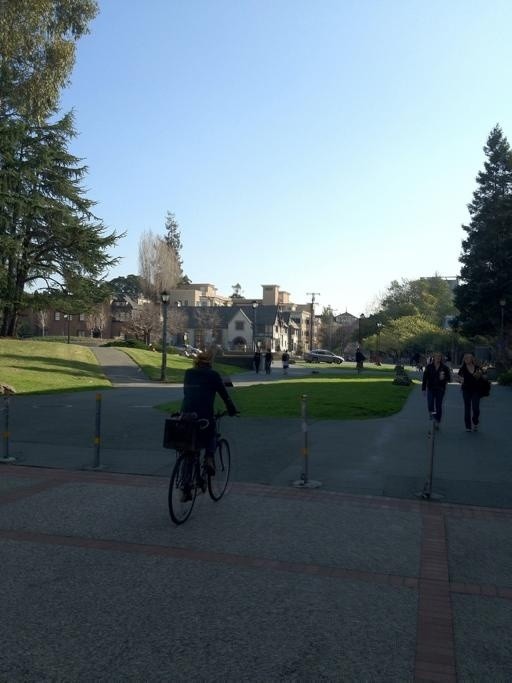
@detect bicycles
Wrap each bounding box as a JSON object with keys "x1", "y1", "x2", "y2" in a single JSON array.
[{"x1": 167, "y1": 408, "x2": 242, "y2": 525}]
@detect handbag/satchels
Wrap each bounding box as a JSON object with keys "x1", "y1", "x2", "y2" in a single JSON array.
[{"x1": 478, "y1": 378, "x2": 490, "y2": 397}]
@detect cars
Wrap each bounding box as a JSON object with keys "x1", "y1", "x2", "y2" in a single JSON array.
[
  {"x1": 303, "y1": 349, "x2": 344, "y2": 364},
  {"x1": 179, "y1": 344, "x2": 203, "y2": 354}
]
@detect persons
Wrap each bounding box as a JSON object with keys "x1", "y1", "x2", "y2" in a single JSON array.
[
  {"x1": 458, "y1": 354, "x2": 490, "y2": 432},
  {"x1": 356, "y1": 348, "x2": 365, "y2": 368},
  {"x1": 180, "y1": 352, "x2": 237, "y2": 503},
  {"x1": 255, "y1": 349, "x2": 273, "y2": 376},
  {"x1": 422, "y1": 352, "x2": 450, "y2": 423},
  {"x1": 282, "y1": 350, "x2": 289, "y2": 375}
]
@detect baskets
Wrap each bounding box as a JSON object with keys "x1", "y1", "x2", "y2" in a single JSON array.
[{"x1": 163, "y1": 414, "x2": 199, "y2": 449}]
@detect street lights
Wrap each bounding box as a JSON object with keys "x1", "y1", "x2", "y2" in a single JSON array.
[
  {"x1": 377, "y1": 323, "x2": 382, "y2": 360},
  {"x1": 161, "y1": 290, "x2": 170, "y2": 381},
  {"x1": 252, "y1": 300, "x2": 258, "y2": 353},
  {"x1": 67, "y1": 288, "x2": 72, "y2": 344}
]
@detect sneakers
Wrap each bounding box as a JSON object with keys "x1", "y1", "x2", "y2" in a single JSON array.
[
  {"x1": 181, "y1": 491, "x2": 193, "y2": 503},
  {"x1": 466, "y1": 425, "x2": 479, "y2": 432},
  {"x1": 204, "y1": 457, "x2": 216, "y2": 476}
]
